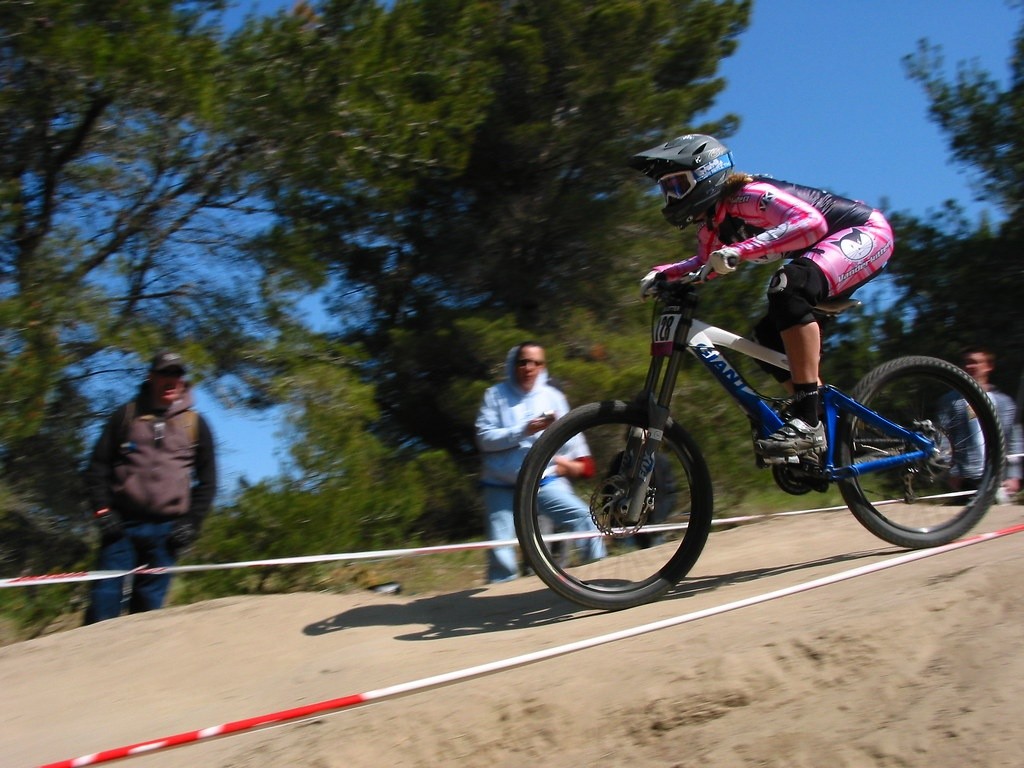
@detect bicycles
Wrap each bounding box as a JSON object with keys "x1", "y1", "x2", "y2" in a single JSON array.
[{"x1": 514, "y1": 253, "x2": 1008, "y2": 613}]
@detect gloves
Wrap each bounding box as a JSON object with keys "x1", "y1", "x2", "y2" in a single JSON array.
[
  {"x1": 638, "y1": 270, "x2": 667, "y2": 303},
  {"x1": 95, "y1": 510, "x2": 119, "y2": 538},
  {"x1": 168, "y1": 520, "x2": 201, "y2": 545},
  {"x1": 698, "y1": 246, "x2": 740, "y2": 288}
]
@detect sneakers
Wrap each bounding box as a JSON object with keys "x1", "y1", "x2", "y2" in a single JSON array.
[{"x1": 754, "y1": 418, "x2": 827, "y2": 457}]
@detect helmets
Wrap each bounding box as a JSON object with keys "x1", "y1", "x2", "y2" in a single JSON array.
[{"x1": 641, "y1": 134, "x2": 735, "y2": 227}]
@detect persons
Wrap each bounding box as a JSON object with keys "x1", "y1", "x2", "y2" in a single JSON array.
[
  {"x1": 937, "y1": 345, "x2": 1022, "y2": 505},
  {"x1": 475, "y1": 340, "x2": 606, "y2": 584},
  {"x1": 630, "y1": 134, "x2": 893, "y2": 492},
  {"x1": 85, "y1": 350, "x2": 216, "y2": 626}
]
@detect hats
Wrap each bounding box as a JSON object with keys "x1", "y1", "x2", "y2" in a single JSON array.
[{"x1": 150, "y1": 350, "x2": 187, "y2": 376}]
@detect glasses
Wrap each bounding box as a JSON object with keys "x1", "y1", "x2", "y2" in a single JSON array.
[
  {"x1": 516, "y1": 358, "x2": 545, "y2": 367},
  {"x1": 151, "y1": 421, "x2": 166, "y2": 450},
  {"x1": 658, "y1": 170, "x2": 699, "y2": 207}
]
